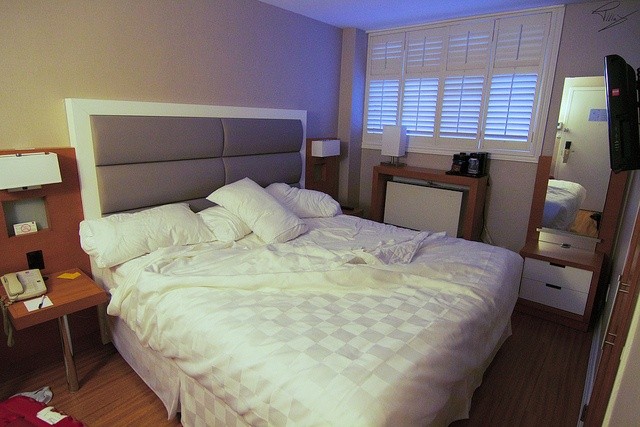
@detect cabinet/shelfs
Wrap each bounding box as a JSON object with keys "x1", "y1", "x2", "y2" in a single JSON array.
[
  {"x1": 369, "y1": 164, "x2": 490, "y2": 241},
  {"x1": 515, "y1": 254, "x2": 608, "y2": 333}
]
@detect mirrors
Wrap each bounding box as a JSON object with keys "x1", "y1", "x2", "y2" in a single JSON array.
[{"x1": 541, "y1": 74, "x2": 637, "y2": 239}]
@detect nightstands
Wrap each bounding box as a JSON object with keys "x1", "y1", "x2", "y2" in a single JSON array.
[
  {"x1": 0, "y1": 148, "x2": 109, "y2": 394},
  {"x1": 306, "y1": 138, "x2": 365, "y2": 217}
]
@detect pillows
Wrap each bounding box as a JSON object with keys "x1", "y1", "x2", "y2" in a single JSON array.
[
  {"x1": 264, "y1": 182, "x2": 344, "y2": 218},
  {"x1": 196, "y1": 205, "x2": 253, "y2": 244},
  {"x1": 79, "y1": 202, "x2": 218, "y2": 270},
  {"x1": 205, "y1": 174, "x2": 309, "y2": 244}
]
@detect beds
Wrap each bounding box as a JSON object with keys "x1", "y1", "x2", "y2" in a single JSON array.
[
  {"x1": 64, "y1": 97, "x2": 524, "y2": 427},
  {"x1": 541, "y1": 179, "x2": 587, "y2": 231}
]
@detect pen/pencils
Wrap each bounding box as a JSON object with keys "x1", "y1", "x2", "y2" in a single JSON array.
[{"x1": 39, "y1": 295, "x2": 45, "y2": 308}]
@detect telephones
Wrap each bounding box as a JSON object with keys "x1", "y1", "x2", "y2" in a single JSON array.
[{"x1": 0, "y1": 269, "x2": 47, "y2": 302}]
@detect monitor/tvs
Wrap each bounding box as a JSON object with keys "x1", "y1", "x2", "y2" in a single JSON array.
[{"x1": 603, "y1": 52, "x2": 640, "y2": 175}]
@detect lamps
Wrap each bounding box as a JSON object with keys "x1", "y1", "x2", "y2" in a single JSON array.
[
  {"x1": 311, "y1": 140, "x2": 342, "y2": 159},
  {"x1": 380, "y1": 123, "x2": 407, "y2": 167},
  {"x1": 0, "y1": 150, "x2": 64, "y2": 194}
]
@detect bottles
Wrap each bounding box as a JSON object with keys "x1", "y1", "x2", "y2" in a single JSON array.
[
  {"x1": 459, "y1": 152, "x2": 466, "y2": 172},
  {"x1": 452, "y1": 154, "x2": 462, "y2": 174},
  {"x1": 467, "y1": 153, "x2": 479, "y2": 174}
]
